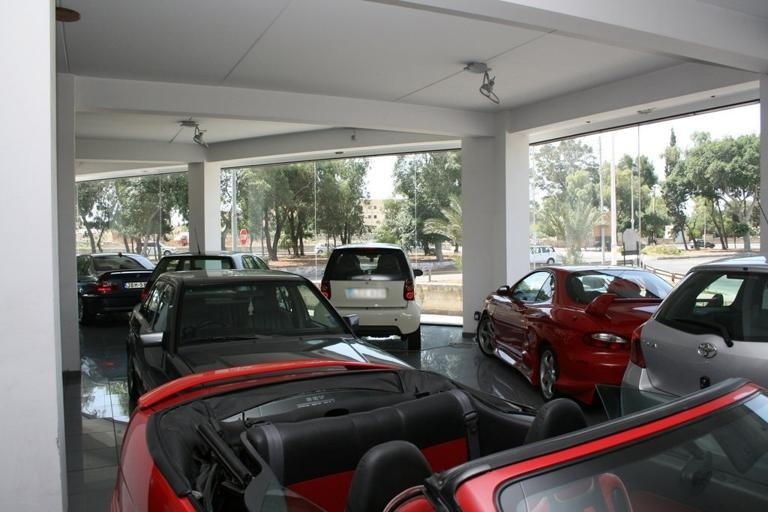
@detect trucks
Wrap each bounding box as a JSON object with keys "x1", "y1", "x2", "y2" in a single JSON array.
[{"x1": 174, "y1": 232, "x2": 189, "y2": 247}]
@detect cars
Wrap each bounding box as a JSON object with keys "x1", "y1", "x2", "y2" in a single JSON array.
[
  {"x1": 315, "y1": 242, "x2": 342, "y2": 254},
  {"x1": 140, "y1": 251, "x2": 271, "y2": 306},
  {"x1": 141, "y1": 240, "x2": 176, "y2": 257},
  {"x1": 476, "y1": 264, "x2": 675, "y2": 407},
  {"x1": 76, "y1": 251, "x2": 157, "y2": 329},
  {"x1": 618, "y1": 250, "x2": 768, "y2": 458},
  {"x1": 689, "y1": 239, "x2": 715, "y2": 249},
  {"x1": 530, "y1": 245, "x2": 560, "y2": 265},
  {"x1": 313, "y1": 242, "x2": 424, "y2": 352},
  {"x1": 124, "y1": 268, "x2": 418, "y2": 404}
]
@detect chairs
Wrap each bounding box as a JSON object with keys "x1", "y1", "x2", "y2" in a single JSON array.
[
  {"x1": 119, "y1": 260, "x2": 133, "y2": 270},
  {"x1": 350, "y1": 438, "x2": 442, "y2": 512},
  {"x1": 178, "y1": 296, "x2": 216, "y2": 341},
  {"x1": 244, "y1": 295, "x2": 293, "y2": 331},
  {"x1": 607, "y1": 277, "x2": 642, "y2": 298},
  {"x1": 511, "y1": 395, "x2": 636, "y2": 512},
  {"x1": 339, "y1": 254, "x2": 397, "y2": 274}
]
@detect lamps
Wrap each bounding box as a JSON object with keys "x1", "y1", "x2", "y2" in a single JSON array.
[
  {"x1": 179, "y1": 120, "x2": 208, "y2": 149},
  {"x1": 465, "y1": 61, "x2": 502, "y2": 105}
]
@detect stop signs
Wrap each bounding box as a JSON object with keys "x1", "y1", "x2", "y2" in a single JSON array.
[{"x1": 240, "y1": 228, "x2": 248, "y2": 245}]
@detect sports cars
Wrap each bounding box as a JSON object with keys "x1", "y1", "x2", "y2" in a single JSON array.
[{"x1": 109, "y1": 359, "x2": 768, "y2": 512}]
